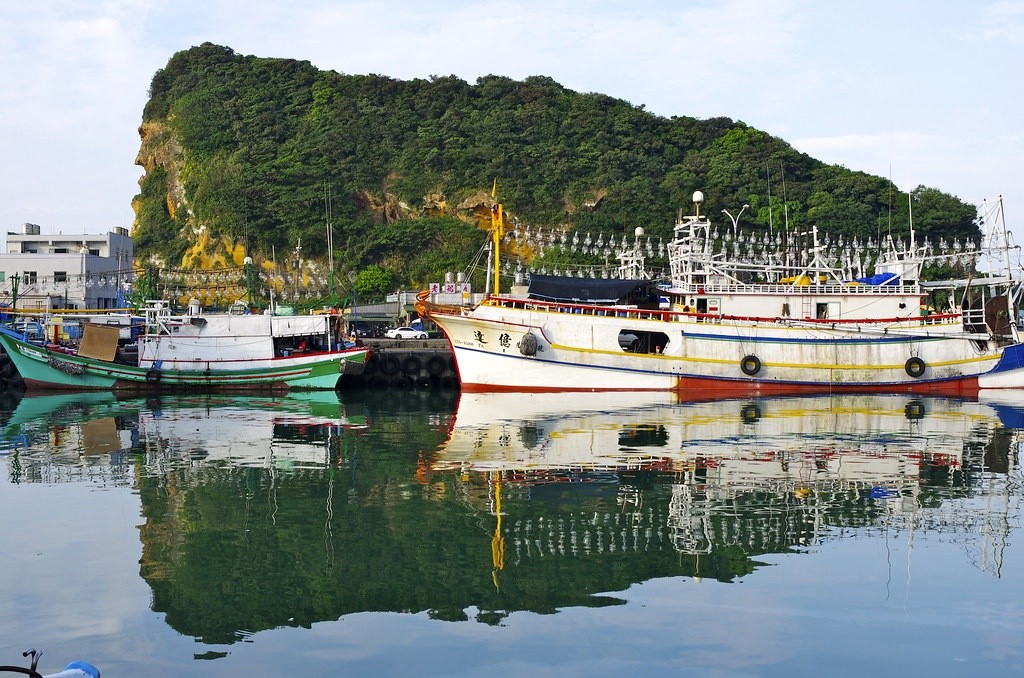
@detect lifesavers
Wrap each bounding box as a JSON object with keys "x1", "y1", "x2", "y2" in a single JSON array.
[
  {"x1": 146, "y1": 370, "x2": 161, "y2": 384},
  {"x1": 365, "y1": 355, "x2": 379, "y2": 374},
  {"x1": 403, "y1": 356, "x2": 421, "y2": 374},
  {"x1": 905, "y1": 357, "x2": 925, "y2": 377},
  {"x1": 442, "y1": 376, "x2": 453, "y2": 382},
  {"x1": 740, "y1": 355, "x2": 761, "y2": 375},
  {"x1": 426, "y1": 356, "x2": 445, "y2": 376},
  {"x1": 740, "y1": 406, "x2": 761, "y2": 425},
  {"x1": 380, "y1": 356, "x2": 400, "y2": 374},
  {"x1": 449, "y1": 356, "x2": 456, "y2": 373},
  {"x1": 905, "y1": 401, "x2": 925, "y2": 419},
  {"x1": 395, "y1": 377, "x2": 414, "y2": 392},
  {"x1": 414, "y1": 377, "x2": 436, "y2": 388}
]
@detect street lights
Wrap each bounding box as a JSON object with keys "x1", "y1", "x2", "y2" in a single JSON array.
[{"x1": 484, "y1": 242, "x2": 493, "y2": 298}]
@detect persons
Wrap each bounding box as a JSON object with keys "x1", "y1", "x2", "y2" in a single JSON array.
[
  {"x1": 697, "y1": 306, "x2": 706, "y2": 319},
  {"x1": 299, "y1": 340, "x2": 309, "y2": 357}
]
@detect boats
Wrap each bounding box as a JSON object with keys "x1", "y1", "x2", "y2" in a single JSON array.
[
  {"x1": 0, "y1": 390, "x2": 375, "y2": 573},
  {"x1": 0, "y1": 258, "x2": 374, "y2": 390},
  {"x1": 412, "y1": 173, "x2": 1023, "y2": 384},
  {"x1": 418, "y1": 383, "x2": 1023, "y2": 590}
]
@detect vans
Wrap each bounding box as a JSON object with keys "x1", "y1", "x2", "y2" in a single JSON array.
[{"x1": 5, "y1": 321, "x2": 40, "y2": 338}]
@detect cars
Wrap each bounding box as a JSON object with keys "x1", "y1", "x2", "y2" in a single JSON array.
[
  {"x1": 386, "y1": 325, "x2": 430, "y2": 340},
  {"x1": 351, "y1": 329, "x2": 363, "y2": 337}
]
